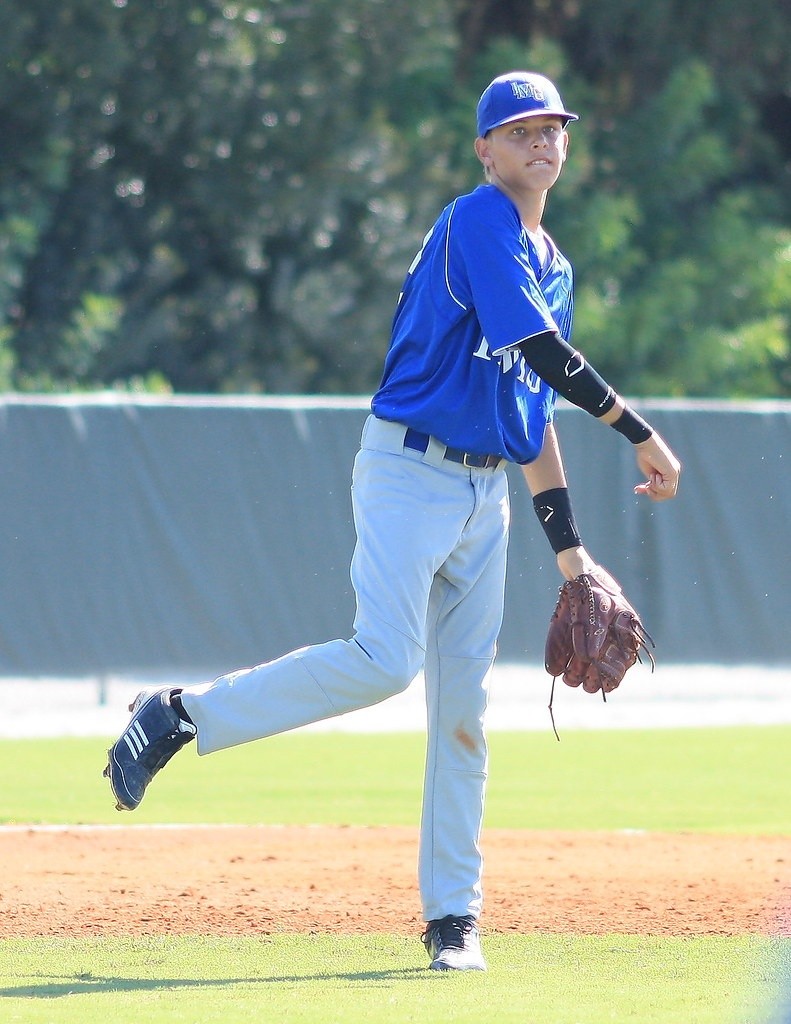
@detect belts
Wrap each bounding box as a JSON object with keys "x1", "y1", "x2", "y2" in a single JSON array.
[{"x1": 403, "y1": 428, "x2": 503, "y2": 469}]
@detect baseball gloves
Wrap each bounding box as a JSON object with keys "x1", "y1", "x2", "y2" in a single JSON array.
[{"x1": 542, "y1": 564, "x2": 658, "y2": 743}]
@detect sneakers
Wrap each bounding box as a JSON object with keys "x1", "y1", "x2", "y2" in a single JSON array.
[
  {"x1": 103, "y1": 685, "x2": 198, "y2": 812},
  {"x1": 419, "y1": 914, "x2": 487, "y2": 971}
]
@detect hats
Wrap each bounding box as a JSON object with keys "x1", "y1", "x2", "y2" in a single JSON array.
[{"x1": 475, "y1": 71, "x2": 580, "y2": 139}]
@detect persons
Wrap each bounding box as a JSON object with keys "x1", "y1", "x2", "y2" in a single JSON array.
[{"x1": 104, "y1": 71, "x2": 680, "y2": 971}]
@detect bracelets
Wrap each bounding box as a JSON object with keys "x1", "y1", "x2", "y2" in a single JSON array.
[
  {"x1": 610, "y1": 403, "x2": 654, "y2": 445},
  {"x1": 533, "y1": 488, "x2": 581, "y2": 554}
]
